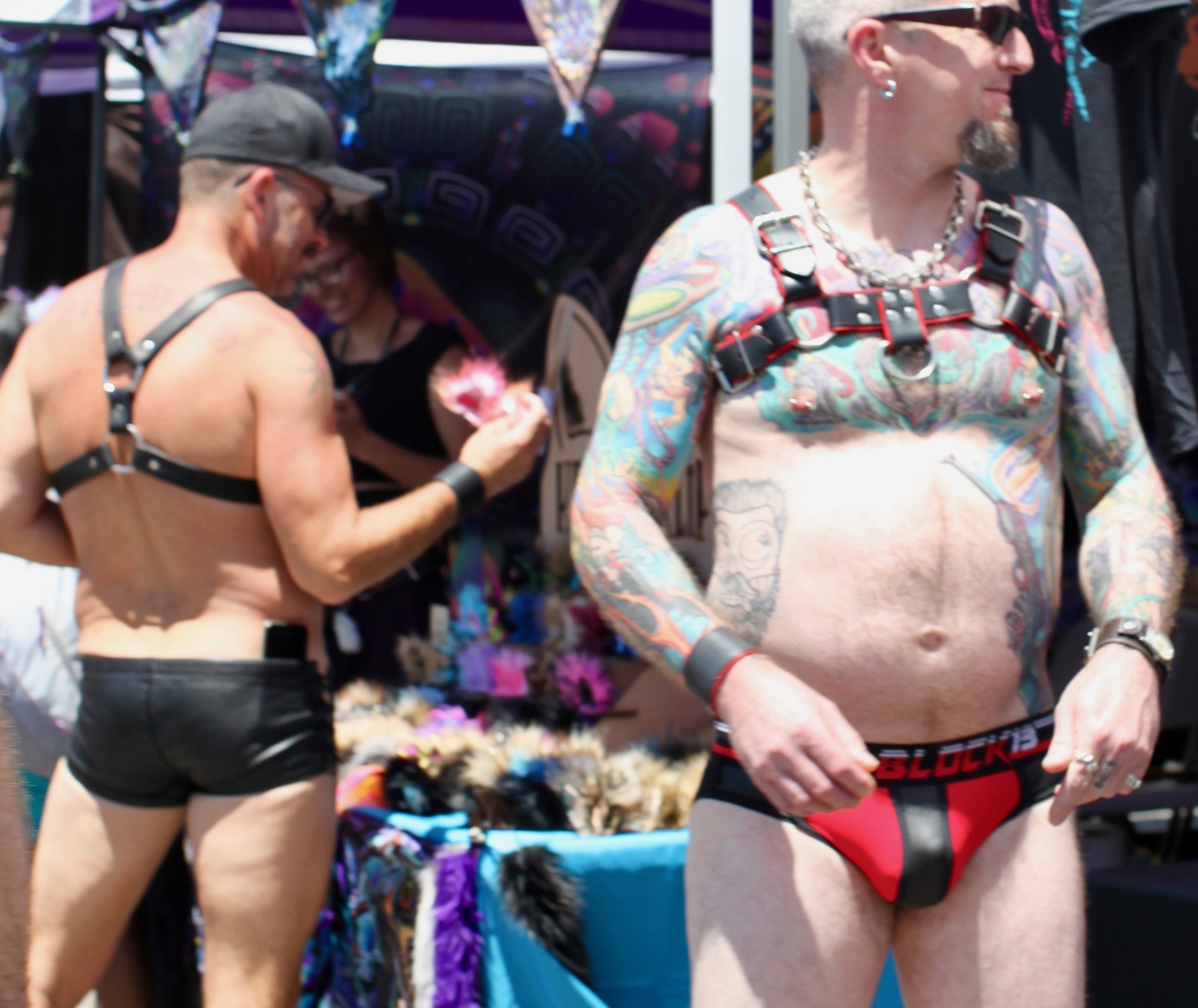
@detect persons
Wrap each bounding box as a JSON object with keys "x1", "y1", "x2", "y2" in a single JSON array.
[
  {"x1": 301, "y1": 198, "x2": 479, "y2": 683},
  {"x1": 0, "y1": 82, "x2": 552, "y2": 1008},
  {"x1": 568, "y1": 0, "x2": 1185, "y2": 1008},
  {"x1": 1, "y1": 164, "x2": 32, "y2": 374}
]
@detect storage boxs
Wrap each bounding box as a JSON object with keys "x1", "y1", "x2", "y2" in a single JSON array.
[{"x1": 295, "y1": 804, "x2": 906, "y2": 1008}]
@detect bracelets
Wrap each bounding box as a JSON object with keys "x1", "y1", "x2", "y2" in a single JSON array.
[
  {"x1": 683, "y1": 629, "x2": 761, "y2": 710},
  {"x1": 1084, "y1": 618, "x2": 1175, "y2": 684},
  {"x1": 433, "y1": 460, "x2": 486, "y2": 527}
]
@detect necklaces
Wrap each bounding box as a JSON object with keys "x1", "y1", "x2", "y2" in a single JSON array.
[{"x1": 798, "y1": 145, "x2": 968, "y2": 290}]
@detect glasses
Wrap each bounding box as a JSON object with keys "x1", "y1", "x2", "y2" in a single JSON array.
[
  {"x1": 842, "y1": 4, "x2": 1026, "y2": 45},
  {"x1": 233, "y1": 167, "x2": 334, "y2": 228},
  {"x1": 299, "y1": 247, "x2": 361, "y2": 289}
]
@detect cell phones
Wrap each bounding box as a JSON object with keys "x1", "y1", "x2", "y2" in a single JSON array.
[{"x1": 262, "y1": 620, "x2": 309, "y2": 661}]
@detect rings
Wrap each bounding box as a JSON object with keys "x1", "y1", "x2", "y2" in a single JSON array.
[
  {"x1": 1073, "y1": 750, "x2": 1099, "y2": 773},
  {"x1": 1125, "y1": 772, "x2": 1143, "y2": 789}
]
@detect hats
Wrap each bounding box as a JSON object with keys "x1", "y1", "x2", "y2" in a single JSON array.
[{"x1": 178, "y1": 80, "x2": 386, "y2": 212}]
